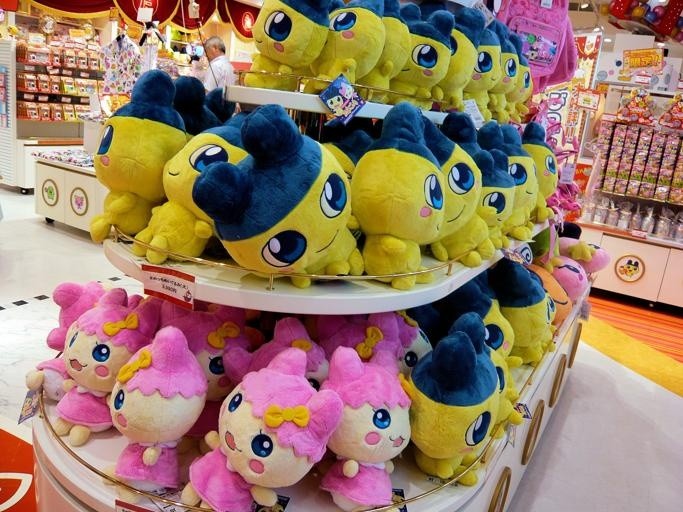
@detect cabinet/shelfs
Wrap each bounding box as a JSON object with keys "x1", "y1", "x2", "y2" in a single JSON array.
[
  {"x1": 1, "y1": 34, "x2": 105, "y2": 196},
  {"x1": 30, "y1": 69, "x2": 598, "y2": 511}
]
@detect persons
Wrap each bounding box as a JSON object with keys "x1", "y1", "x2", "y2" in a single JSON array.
[{"x1": 203, "y1": 36, "x2": 236, "y2": 95}]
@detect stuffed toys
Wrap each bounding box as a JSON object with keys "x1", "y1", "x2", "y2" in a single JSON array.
[
  {"x1": 25, "y1": 237, "x2": 609, "y2": 511},
  {"x1": 24, "y1": 1, "x2": 610, "y2": 511},
  {"x1": 246, "y1": 0, "x2": 533, "y2": 126},
  {"x1": 90, "y1": 70, "x2": 581, "y2": 290}
]
